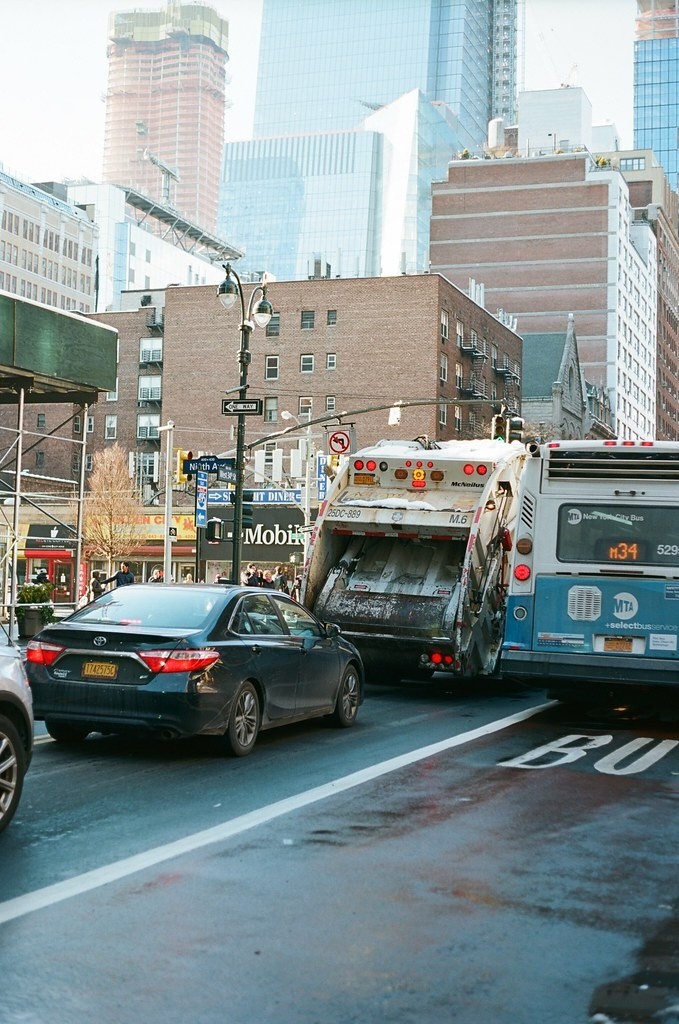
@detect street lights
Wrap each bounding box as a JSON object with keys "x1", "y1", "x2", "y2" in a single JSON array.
[
  {"x1": 279, "y1": 406, "x2": 314, "y2": 565},
  {"x1": 218, "y1": 261, "x2": 274, "y2": 585}
]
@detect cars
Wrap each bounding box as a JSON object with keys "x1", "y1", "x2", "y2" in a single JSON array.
[
  {"x1": 0, "y1": 645, "x2": 34, "y2": 835},
  {"x1": 26, "y1": 580, "x2": 368, "y2": 758}
]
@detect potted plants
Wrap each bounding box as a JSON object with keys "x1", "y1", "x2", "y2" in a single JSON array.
[{"x1": 14, "y1": 581, "x2": 58, "y2": 639}]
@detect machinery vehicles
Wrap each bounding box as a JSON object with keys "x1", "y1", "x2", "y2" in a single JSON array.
[{"x1": 295, "y1": 430, "x2": 537, "y2": 687}]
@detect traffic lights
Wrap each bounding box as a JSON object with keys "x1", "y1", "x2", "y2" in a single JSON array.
[
  {"x1": 330, "y1": 454, "x2": 340, "y2": 468},
  {"x1": 176, "y1": 449, "x2": 193, "y2": 485},
  {"x1": 242, "y1": 489, "x2": 255, "y2": 529},
  {"x1": 491, "y1": 414, "x2": 504, "y2": 441},
  {"x1": 506, "y1": 417, "x2": 522, "y2": 444},
  {"x1": 207, "y1": 517, "x2": 224, "y2": 543}
]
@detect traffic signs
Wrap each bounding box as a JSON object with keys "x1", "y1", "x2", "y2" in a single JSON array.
[
  {"x1": 181, "y1": 455, "x2": 235, "y2": 475},
  {"x1": 221, "y1": 397, "x2": 260, "y2": 415},
  {"x1": 216, "y1": 466, "x2": 239, "y2": 484}
]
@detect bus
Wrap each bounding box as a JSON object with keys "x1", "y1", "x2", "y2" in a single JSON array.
[{"x1": 497, "y1": 437, "x2": 679, "y2": 709}]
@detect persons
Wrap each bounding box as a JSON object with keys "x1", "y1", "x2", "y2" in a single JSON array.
[
  {"x1": 90, "y1": 570, "x2": 106, "y2": 601},
  {"x1": 99, "y1": 561, "x2": 135, "y2": 588},
  {"x1": 147, "y1": 562, "x2": 305, "y2": 604}
]
